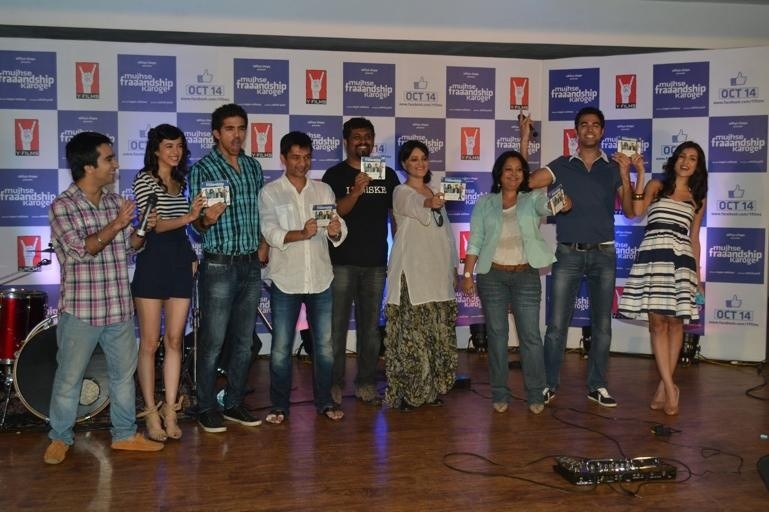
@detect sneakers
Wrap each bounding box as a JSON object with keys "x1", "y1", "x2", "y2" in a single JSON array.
[
  {"x1": 493, "y1": 403, "x2": 508, "y2": 413},
  {"x1": 111, "y1": 432, "x2": 164, "y2": 452},
  {"x1": 322, "y1": 381, "x2": 384, "y2": 421},
  {"x1": 587, "y1": 387, "x2": 618, "y2": 408},
  {"x1": 196, "y1": 402, "x2": 287, "y2": 433},
  {"x1": 44, "y1": 440, "x2": 68, "y2": 466},
  {"x1": 530, "y1": 386, "x2": 555, "y2": 413}
]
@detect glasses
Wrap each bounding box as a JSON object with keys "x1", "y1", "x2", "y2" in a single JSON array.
[{"x1": 432, "y1": 206, "x2": 444, "y2": 227}]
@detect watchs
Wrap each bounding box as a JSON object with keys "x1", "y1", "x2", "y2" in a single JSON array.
[{"x1": 464, "y1": 272, "x2": 472, "y2": 278}]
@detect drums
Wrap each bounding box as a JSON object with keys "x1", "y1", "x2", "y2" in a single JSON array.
[
  {"x1": 13, "y1": 315, "x2": 111, "y2": 425},
  {"x1": 0, "y1": 289, "x2": 48, "y2": 364}
]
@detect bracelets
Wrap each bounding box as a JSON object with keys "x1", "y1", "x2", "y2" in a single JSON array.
[
  {"x1": 95, "y1": 232, "x2": 106, "y2": 246},
  {"x1": 633, "y1": 194, "x2": 646, "y2": 199}
]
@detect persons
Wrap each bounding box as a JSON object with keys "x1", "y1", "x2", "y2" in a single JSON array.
[
  {"x1": 364, "y1": 162, "x2": 383, "y2": 172},
  {"x1": 316, "y1": 209, "x2": 334, "y2": 220},
  {"x1": 553, "y1": 191, "x2": 564, "y2": 206},
  {"x1": 322, "y1": 117, "x2": 402, "y2": 407},
  {"x1": 461, "y1": 151, "x2": 573, "y2": 415},
  {"x1": 132, "y1": 123, "x2": 207, "y2": 443},
  {"x1": 188, "y1": 103, "x2": 269, "y2": 432},
  {"x1": 444, "y1": 183, "x2": 464, "y2": 193},
  {"x1": 631, "y1": 142, "x2": 707, "y2": 415},
  {"x1": 621, "y1": 142, "x2": 638, "y2": 151},
  {"x1": 520, "y1": 107, "x2": 635, "y2": 408},
  {"x1": 206, "y1": 187, "x2": 227, "y2": 198},
  {"x1": 257, "y1": 131, "x2": 345, "y2": 425},
  {"x1": 381, "y1": 140, "x2": 460, "y2": 410},
  {"x1": 45, "y1": 132, "x2": 165, "y2": 465}
]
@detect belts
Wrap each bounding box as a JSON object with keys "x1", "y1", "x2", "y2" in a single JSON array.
[
  {"x1": 564, "y1": 241, "x2": 596, "y2": 252},
  {"x1": 493, "y1": 261, "x2": 528, "y2": 271},
  {"x1": 202, "y1": 250, "x2": 256, "y2": 262}
]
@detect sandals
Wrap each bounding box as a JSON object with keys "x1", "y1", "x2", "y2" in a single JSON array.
[{"x1": 141, "y1": 404, "x2": 181, "y2": 440}]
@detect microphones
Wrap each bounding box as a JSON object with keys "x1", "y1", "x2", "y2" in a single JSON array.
[
  {"x1": 135, "y1": 193, "x2": 158, "y2": 237},
  {"x1": 517, "y1": 115, "x2": 539, "y2": 137}
]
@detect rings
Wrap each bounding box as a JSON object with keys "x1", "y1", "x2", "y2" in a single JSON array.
[{"x1": 361, "y1": 175, "x2": 364, "y2": 179}]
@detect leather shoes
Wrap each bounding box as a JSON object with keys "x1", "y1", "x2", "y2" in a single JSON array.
[
  {"x1": 402, "y1": 401, "x2": 414, "y2": 412},
  {"x1": 431, "y1": 399, "x2": 446, "y2": 406},
  {"x1": 649, "y1": 385, "x2": 680, "y2": 415}
]
